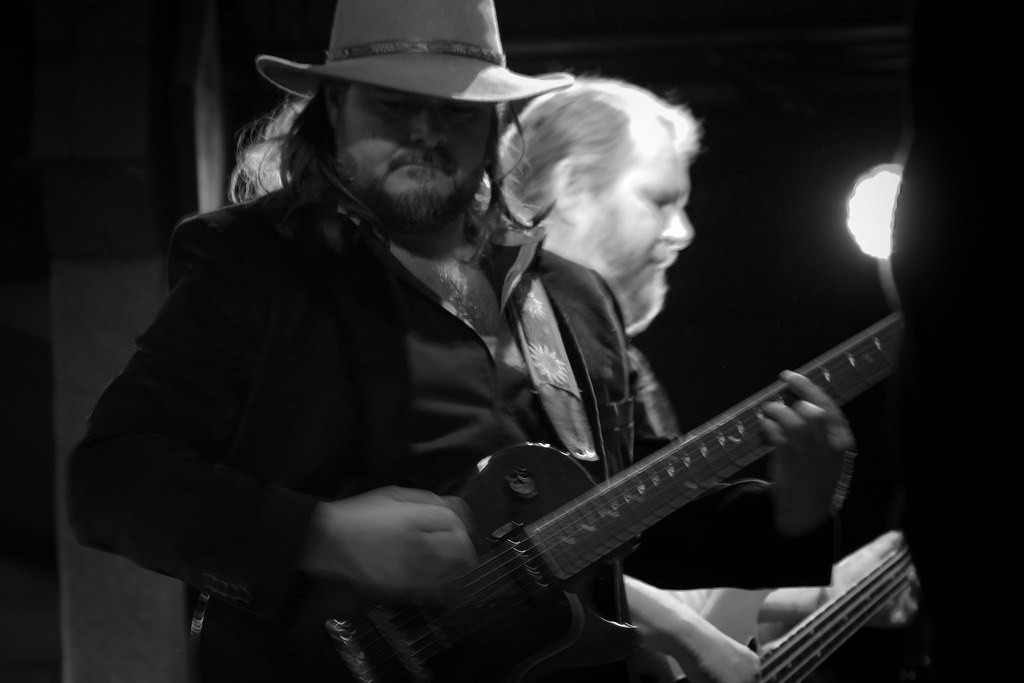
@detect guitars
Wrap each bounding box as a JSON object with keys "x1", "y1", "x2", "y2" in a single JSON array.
[
  {"x1": 181, "y1": 307, "x2": 906, "y2": 681},
  {"x1": 675, "y1": 543, "x2": 920, "y2": 681}
]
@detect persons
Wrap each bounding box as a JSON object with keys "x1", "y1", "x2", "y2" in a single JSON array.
[
  {"x1": 68, "y1": 0, "x2": 856, "y2": 682},
  {"x1": 501, "y1": 78, "x2": 920, "y2": 683}
]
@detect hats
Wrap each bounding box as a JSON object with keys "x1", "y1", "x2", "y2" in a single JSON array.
[{"x1": 255, "y1": 1, "x2": 574, "y2": 103}]
